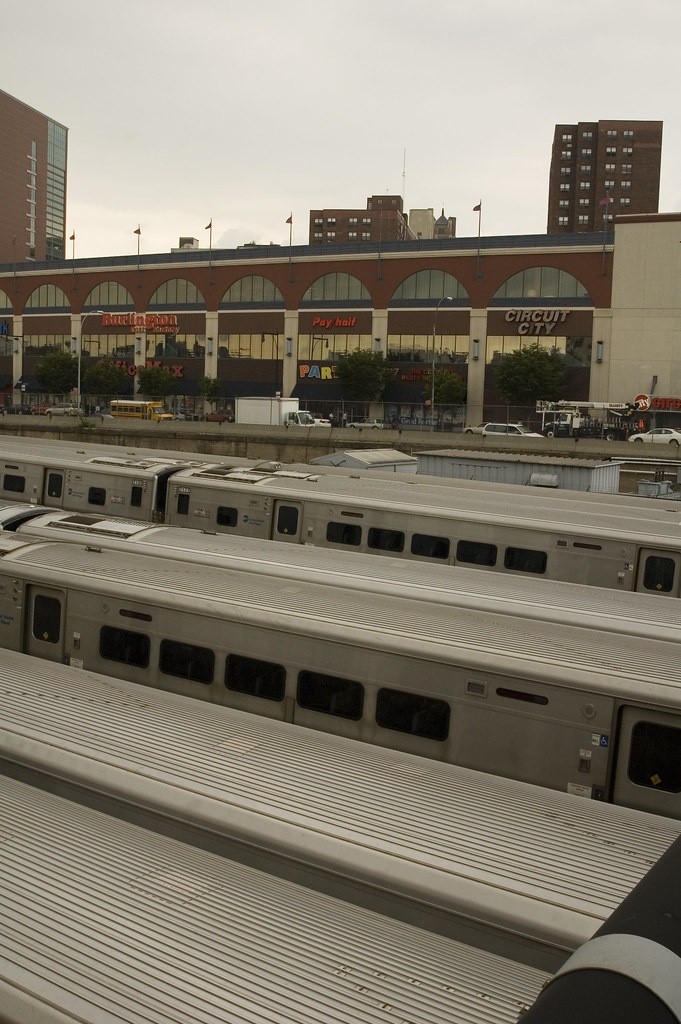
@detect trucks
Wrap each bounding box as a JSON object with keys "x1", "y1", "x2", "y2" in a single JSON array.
[
  {"x1": 235, "y1": 396, "x2": 315, "y2": 427},
  {"x1": 110, "y1": 400, "x2": 174, "y2": 423}
]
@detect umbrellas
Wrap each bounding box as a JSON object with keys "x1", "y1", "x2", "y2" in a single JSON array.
[{"x1": 638, "y1": 415, "x2": 645, "y2": 433}]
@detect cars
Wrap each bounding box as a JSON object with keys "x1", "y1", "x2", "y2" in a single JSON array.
[
  {"x1": 462, "y1": 422, "x2": 490, "y2": 434},
  {"x1": 199, "y1": 410, "x2": 235, "y2": 423},
  {"x1": 45, "y1": 402, "x2": 84, "y2": 417},
  {"x1": 628, "y1": 429, "x2": 681, "y2": 446},
  {"x1": 2, "y1": 404, "x2": 31, "y2": 415},
  {"x1": 346, "y1": 418, "x2": 393, "y2": 430},
  {"x1": 314, "y1": 419, "x2": 331, "y2": 427},
  {"x1": 30, "y1": 403, "x2": 56, "y2": 416},
  {"x1": 172, "y1": 409, "x2": 199, "y2": 422}
]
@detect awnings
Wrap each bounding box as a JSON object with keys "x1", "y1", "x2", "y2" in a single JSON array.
[
  {"x1": 290, "y1": 383, "x2": 368, "y2": 402},
  {"x1": 0, "y1": 375, "x2": 13, "y2": 390},
  {"x1": 379, "y1": 380, "x2": 427, "y2": 404},
  {"x1": 206, "y1": 382, "x2": 282, "y2": 399},
  {"x1": 137, "y1": 380, "x2": 201, "y2": 397},
  {"x1": 80, "y1": 374, "x2": 133, "y2": 395},
  {"x1": 484, "y1": 390, "x2": 535, "y2": 408},
  {"x1": 13, "y1": 374, "x2": 71, "y2": 394}
]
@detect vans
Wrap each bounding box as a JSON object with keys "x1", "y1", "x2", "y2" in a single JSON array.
[{"x1": 482, "y1": 423, "x2": 544, "y2": 438}]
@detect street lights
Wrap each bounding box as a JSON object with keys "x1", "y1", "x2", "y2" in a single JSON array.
[
  {"x1": 77, "y1": 310, "x2": 103, "y2": 408},
  {"x1": 430, "y1": 296, "x2": 452, "y2": 432}
]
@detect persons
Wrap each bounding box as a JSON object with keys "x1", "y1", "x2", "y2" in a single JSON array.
[
  {"x1": 329, "y1": 411, "x2": 334, "y2": 427},
  {"x1": 517, "y1": 418, "x2": 523, "y2": 425},
  {"x1": 343, "y1": 411, "x2": 348, "y2": 428},
  {"x1": 95, "y1": 403, "x2": 100, "y2": 414}
]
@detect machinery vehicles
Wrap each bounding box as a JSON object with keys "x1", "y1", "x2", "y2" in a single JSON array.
[{"x1": 535, "y1": 399, "x2": 640, "y2": 441}]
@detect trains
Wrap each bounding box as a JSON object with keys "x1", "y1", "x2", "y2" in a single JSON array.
[
  {"x1": 0, "y1": 500, "x2": 681, "y2": 822},
  {"x1": 1, "y1": 432, "x2": 681, "y2": 600}
]
